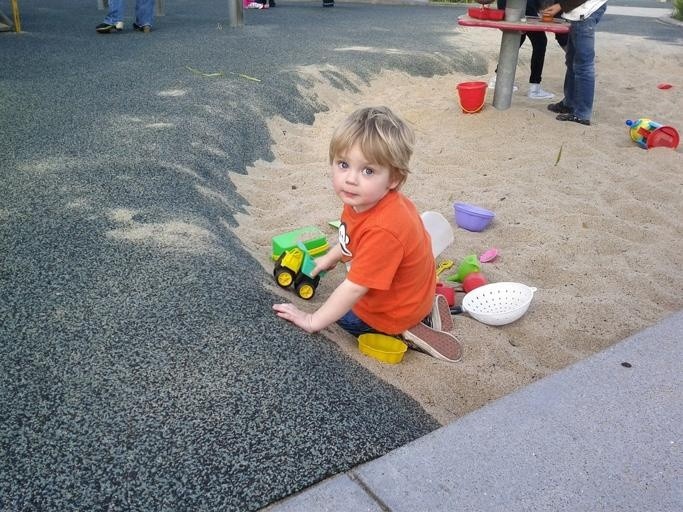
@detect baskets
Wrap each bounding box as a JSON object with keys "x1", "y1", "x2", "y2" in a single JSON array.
[{"x1": 461, "y1": 281, "x2": 538, "y2": 327}]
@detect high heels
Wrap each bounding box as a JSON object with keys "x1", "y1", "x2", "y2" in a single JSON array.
[
  {"x1": 133, "y1": 22, "x2": 150, "y2": 33},
  {"x1": 95, "y1": 21, "x2": 124, "y2": 33}
]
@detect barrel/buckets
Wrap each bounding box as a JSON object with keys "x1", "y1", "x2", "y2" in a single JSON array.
[
  {"x1": 456, "y1": 82, "x2": 487, "y2": 113},
  {"x1": 420, "y1": 211, "x2": 454, "y2": 258}
]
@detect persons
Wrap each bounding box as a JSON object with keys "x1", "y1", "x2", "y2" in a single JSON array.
[
  {"x1": 537, "y1": 1, "x2": 608, "y2": 125},
  {"x1": 269, "y1": 107, "x2": 463, "y2": 363},
  {"x1": 475, "y1": 1, "x2": 555, "y2": 100},
  {"x1": 95, "y1": 0, "x2": 154, "y2": 33}
]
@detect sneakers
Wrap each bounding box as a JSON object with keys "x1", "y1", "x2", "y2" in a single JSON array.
[
  {"x1": 395, "y1": 321, "x2": 463, "y2": 363},
  {"x1": 488, "y1": 77, "x2": 518, "y2": 93},
  {"x1": 424, "y1": 294, "x2": 455, "y2": 332},
  {"x1": 556, "y1": 114, "x2": 590, "y2": 125},
  {"x1": 548, "y1": 101, "x2": 574, "y2": 113},
  {"x1": 527, "y1": 85, "x2": 556, "y2": 100}
]
